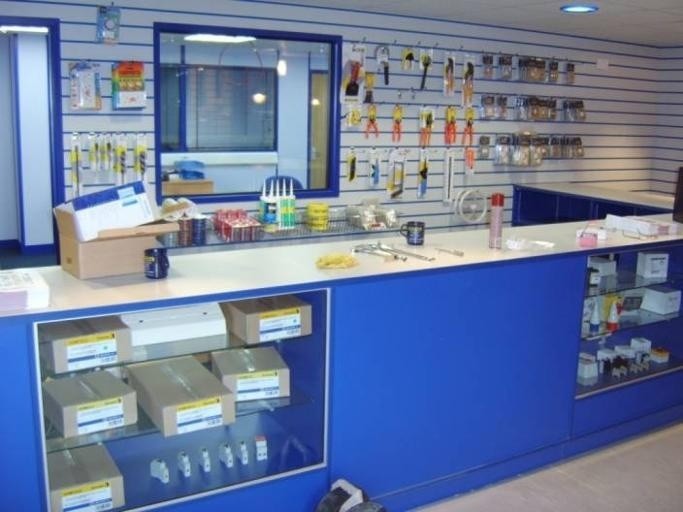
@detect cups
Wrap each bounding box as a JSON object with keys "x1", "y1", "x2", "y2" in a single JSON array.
[
  {"x1": 144, "y1": 248, "x2": 169, "y2": 280},
  {"x1": 400, "y1": 221, "x2": 425, "y2": 246}
]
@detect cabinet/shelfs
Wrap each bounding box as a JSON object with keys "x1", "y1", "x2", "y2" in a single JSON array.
[
  {"x1": 30, "y1": 287, "x2": 331, "y2": 511},
  {"x1": 570, "y1": 243, "x2": 683, "y2": 400}
]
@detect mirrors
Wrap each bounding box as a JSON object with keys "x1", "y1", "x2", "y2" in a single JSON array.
[{"x1": 153, "y1": 22, "x2": 342, "y2": 203}]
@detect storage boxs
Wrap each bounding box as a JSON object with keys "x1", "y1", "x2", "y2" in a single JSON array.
[{"x1": 53, "y1": 198, "x2": 191, "y2": 280}]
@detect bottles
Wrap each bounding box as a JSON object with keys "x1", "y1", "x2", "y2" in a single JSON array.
[{"x1": 489, "y1": 191, "x2": 505, "y2": 249}]
